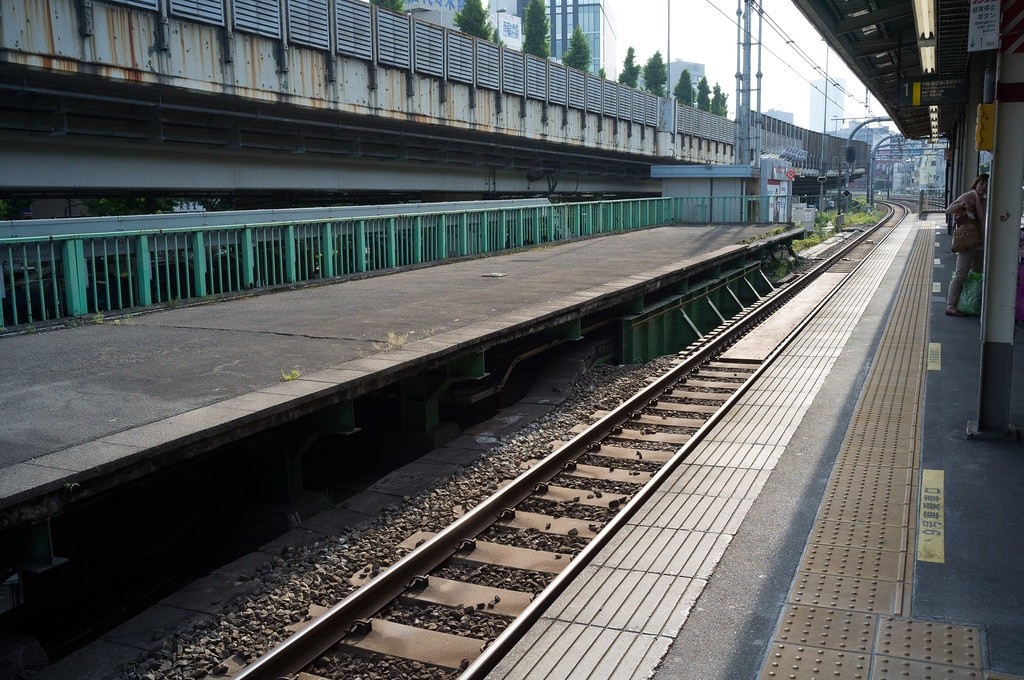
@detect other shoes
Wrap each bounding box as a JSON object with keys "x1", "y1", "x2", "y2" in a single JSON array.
[{"x1": 945, "y1": 309, "x2": 965, "y2": 317}]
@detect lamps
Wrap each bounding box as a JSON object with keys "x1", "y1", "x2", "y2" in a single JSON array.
[
  {"x1": 911, "y1": 0, "x2": 938, "y2": 42},
  {"x1": 919, "y1": 46, "x2": 937, "y2": 75}
]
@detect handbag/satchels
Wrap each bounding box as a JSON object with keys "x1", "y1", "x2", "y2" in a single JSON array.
[{"x1": 951, "y1": 201, "x2": 983, "y2": 252}]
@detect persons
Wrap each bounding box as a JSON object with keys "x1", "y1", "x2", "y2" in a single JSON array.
[{"x1": 945, "y1": 174, "x2": 989, "y2": 316}]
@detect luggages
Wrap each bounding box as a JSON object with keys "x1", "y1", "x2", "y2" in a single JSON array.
[{"x1": 1015, "y1": 257, "x2": 1024, "y2": 324}]
